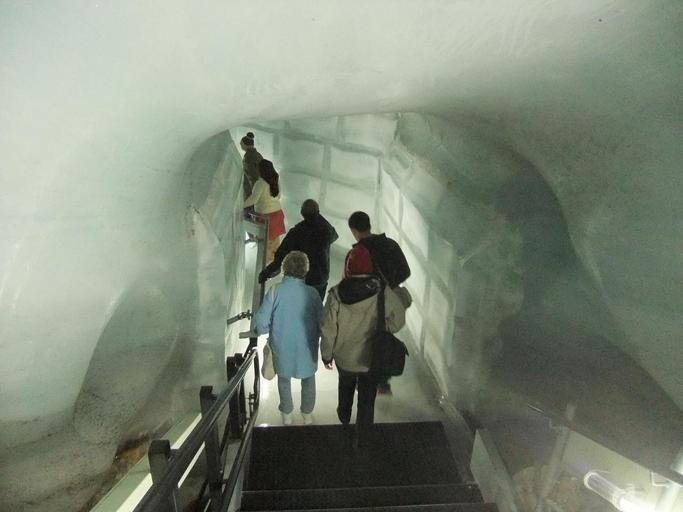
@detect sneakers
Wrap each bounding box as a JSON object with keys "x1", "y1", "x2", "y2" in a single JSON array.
[{"x1": 380, "y1": 383, "x2": 390, "y2": 394}]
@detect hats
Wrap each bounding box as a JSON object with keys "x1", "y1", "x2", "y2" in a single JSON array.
[
  {"x1": 241, "y1": 132, "x2": 254, "y2": 151},
  {"x1": 346, "y1": 245, "x2": 372, "y2": 274}
]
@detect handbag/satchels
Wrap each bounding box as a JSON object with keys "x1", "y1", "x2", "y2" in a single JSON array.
[
  {"x1": 371, "y1": 279, "x2": 409, "y2": 376},
  {"x1": 393, "y1": 287, "x2": 412, "y2": 309},
  {"x1": 261, "y1": 343, "x2": 276, "y2": 380}
]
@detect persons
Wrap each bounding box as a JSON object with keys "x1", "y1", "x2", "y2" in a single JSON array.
[
  {"x1": 240, "y1": 130, "x2": 265, "y2": 218},
  {"x1": 343, "y1": 210, "x2": 412, "y2": 392},
  {"x1": 320, "y1": 245, "x2": 406, "y2": 434},
  {"x1": 244, "y1": 159, "x2": 287, "y2": 267},
  {"x1": 257, "y1": 198, "x2": 338, "y2": 303},
  {"x1": 251, "y1": 250, "x2": 324, "y2": 427}
]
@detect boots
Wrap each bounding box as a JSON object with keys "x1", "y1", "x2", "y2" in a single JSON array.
[
  {"x1": 281, "y1": 411, "x2": 295, "y2": 425},
  {"x1": 301, "y1": 411, "x2": 314, "y2": 425}
]
